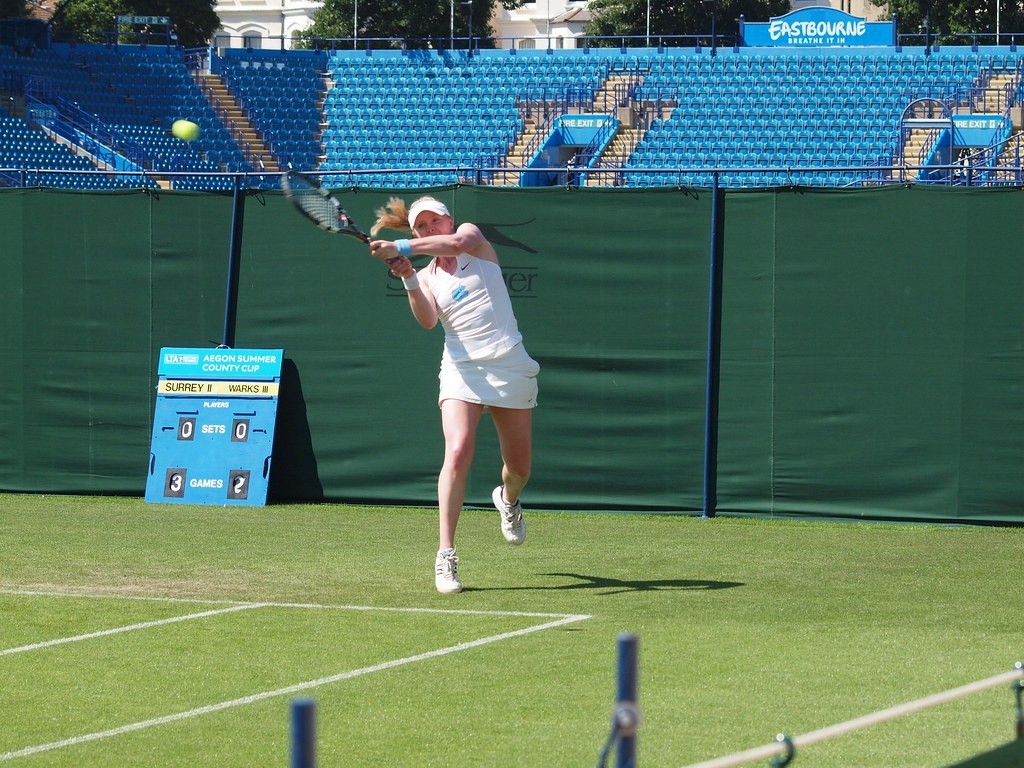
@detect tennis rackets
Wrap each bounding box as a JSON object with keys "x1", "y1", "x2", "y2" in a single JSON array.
[{"x1": 281, "y1": 167, "x2": 400, "y2": 263}]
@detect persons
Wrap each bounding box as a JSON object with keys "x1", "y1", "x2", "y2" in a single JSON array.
[{"x1": 371, "y1": 195, "x2": 540, "y2": 594}]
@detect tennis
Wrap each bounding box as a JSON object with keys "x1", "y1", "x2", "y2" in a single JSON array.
[{"x1": 171, "y1": 118, "x2": 200, "y2": 141}]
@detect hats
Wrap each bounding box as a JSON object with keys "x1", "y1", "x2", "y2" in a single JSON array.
[{"x1": 408, "y1": 200, "x2": 450, "y2": 230}]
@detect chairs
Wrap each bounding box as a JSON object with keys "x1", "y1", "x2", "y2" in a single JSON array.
[{"x1": 0, "y1": 44, "x2": 1024, "y2": 188}]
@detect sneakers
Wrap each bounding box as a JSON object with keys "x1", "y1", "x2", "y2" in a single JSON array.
[
  {"x1": 434, "y1": 545, "x2": 462, "y2": 594},
  {"x1": 492, "y1": 485, "x2": 526, "y2": 545}
]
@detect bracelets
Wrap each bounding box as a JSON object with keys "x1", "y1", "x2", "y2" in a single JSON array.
[{"x1": 394, "y1": 239, "x2": 411, "y2": 256}]
